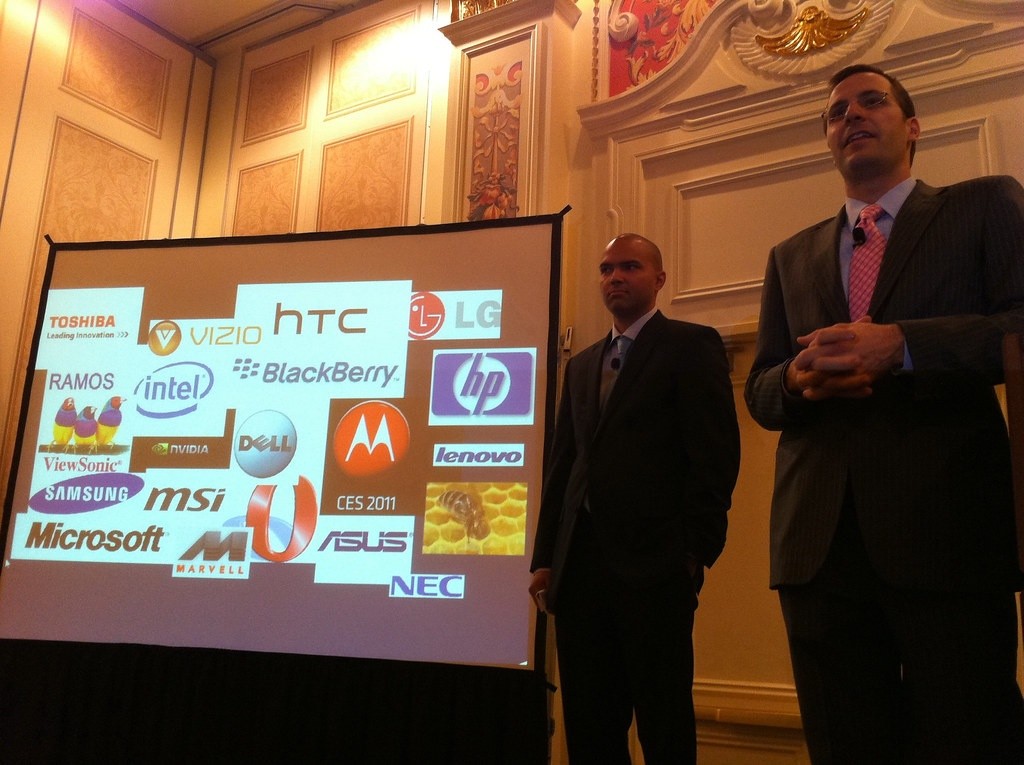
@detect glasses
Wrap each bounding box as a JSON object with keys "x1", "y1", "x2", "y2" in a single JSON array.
[{"x1": 824, "y1": 91, "x2": 898, "y2": 123}]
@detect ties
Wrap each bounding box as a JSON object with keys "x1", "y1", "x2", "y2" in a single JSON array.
[
  {"x1": 847, "y1": 204, "x2": 890, "y2": 322},
  {"x1": 615, "y1": 337, "x2": 633, "y2": 376}
]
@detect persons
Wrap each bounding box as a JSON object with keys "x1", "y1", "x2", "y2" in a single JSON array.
[
  {"x1": 529, "y1": 232, "x2": 742, "y2": 765},
  {"x1": 744, "y1": 63, "x2": 1023, "y2": 765}
]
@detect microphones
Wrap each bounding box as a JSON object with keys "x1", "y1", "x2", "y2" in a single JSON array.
[
  {"x1": 611, "y1": 358, "x2": 621, "y2": 370},
  {"x1": 852, "y1": 228, "x2": 866, "y2": 246}
]
